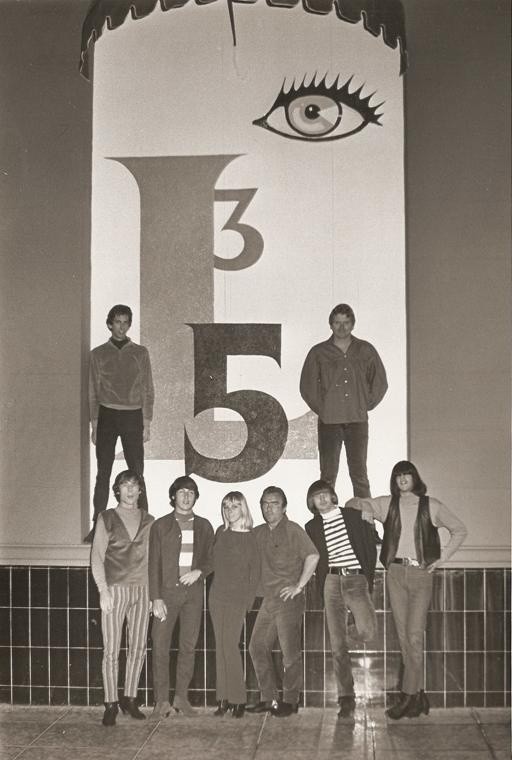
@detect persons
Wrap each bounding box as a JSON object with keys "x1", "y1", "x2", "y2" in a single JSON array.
[
  {"x1": 305, "y1": 480, "x2": 383, "y2": 717},
  {"x1": 90, "y1": 470, "x2": 155, "y2": 726},
  {"x1": 147, "y1": 476, "x2": 215, "y2": 720},
  {"x1": 83, "y1": 304, "x2": 154, "y2": 545},
  {"x1": 208, "y1": 491, "x2": 261, "y2": 719},
  {"x1": 245, "y1": 485, "x2": 321, "y2": 718},
  {"x1": 300, "y1": 304, "x2": 389, "y2": 545},
  {"x1": 345, "y1": 460, "x2": 468, "y2": 718}
]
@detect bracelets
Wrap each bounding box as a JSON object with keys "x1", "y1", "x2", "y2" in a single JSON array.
[{"x1": 295, "y1": 583, "x2": 304, "y2": 590}]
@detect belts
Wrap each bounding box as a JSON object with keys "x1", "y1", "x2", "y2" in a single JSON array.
[
  {"x1": 327, "y1": 568, "x2": 363, "y2": 575},
  {"x1": 392, "y1": 557, "x2": 419, "y2": 567}
]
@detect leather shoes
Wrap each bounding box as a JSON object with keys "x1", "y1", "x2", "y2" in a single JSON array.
[
  {"x1": 103, "y1": 700, "x2": 298, "y2": 727},
  {"x1": 386, "y1": 690, "x2": 429, "y2": 719},
  {"x1": 338, "y1": 697, "x2": 355, "y2": 717}
]
